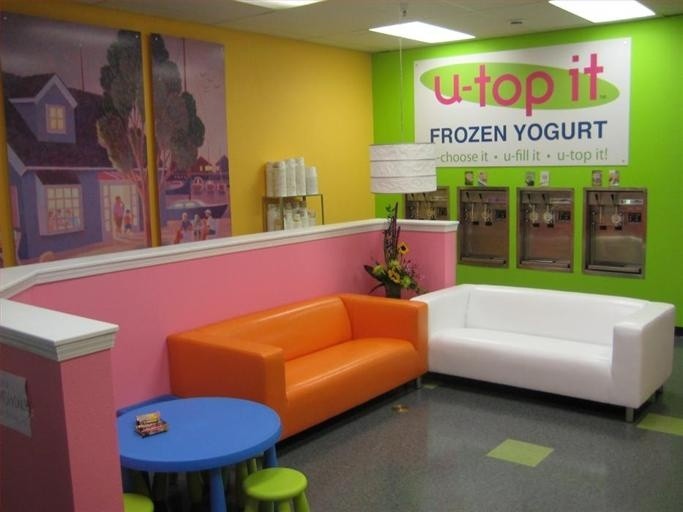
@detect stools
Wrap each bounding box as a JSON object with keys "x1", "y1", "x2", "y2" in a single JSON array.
[{"x1": 241, "y1": 467, "x2": 310, "y2": 511}]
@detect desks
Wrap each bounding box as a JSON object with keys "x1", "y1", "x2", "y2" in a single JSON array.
[{"x1": 115, "y1": 395, "x2": 283, "y2": 511}]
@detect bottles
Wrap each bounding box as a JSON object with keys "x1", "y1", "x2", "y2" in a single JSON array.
[{"x1": 267, "y1": 158, "x2": 320, "y2": 197}]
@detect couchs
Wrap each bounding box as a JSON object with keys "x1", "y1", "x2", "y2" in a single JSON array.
[
  {"x1": 167, "y1": 290, "x2": 429, "y2": 442},
  {"x1": 401, "y1": 282, "x2": 676, "y2": 423}
]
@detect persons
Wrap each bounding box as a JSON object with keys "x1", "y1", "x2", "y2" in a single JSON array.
[
  {"x1": 113, "y1": 196, "x2": 125, "y2": 235},
  {"x1": 123, "y1": 209, "x2": 133, "y2": 233},
  {"x1": 48, "y1": 209, "x2": 79, "y2": 232},
  {"x1": 203, "y1": 209, "x2": 216, "y2": 241},
  {"x1": 193, "y1": 215, "x2": 203, "y2": 241},
  {"x1": 174, "y1": 212, "x2": 193, "y2": 244}
]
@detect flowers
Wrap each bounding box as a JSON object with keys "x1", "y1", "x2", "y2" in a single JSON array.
[{"x1": 359, "y1": 197, "x2": 428, "y2": 296}]
